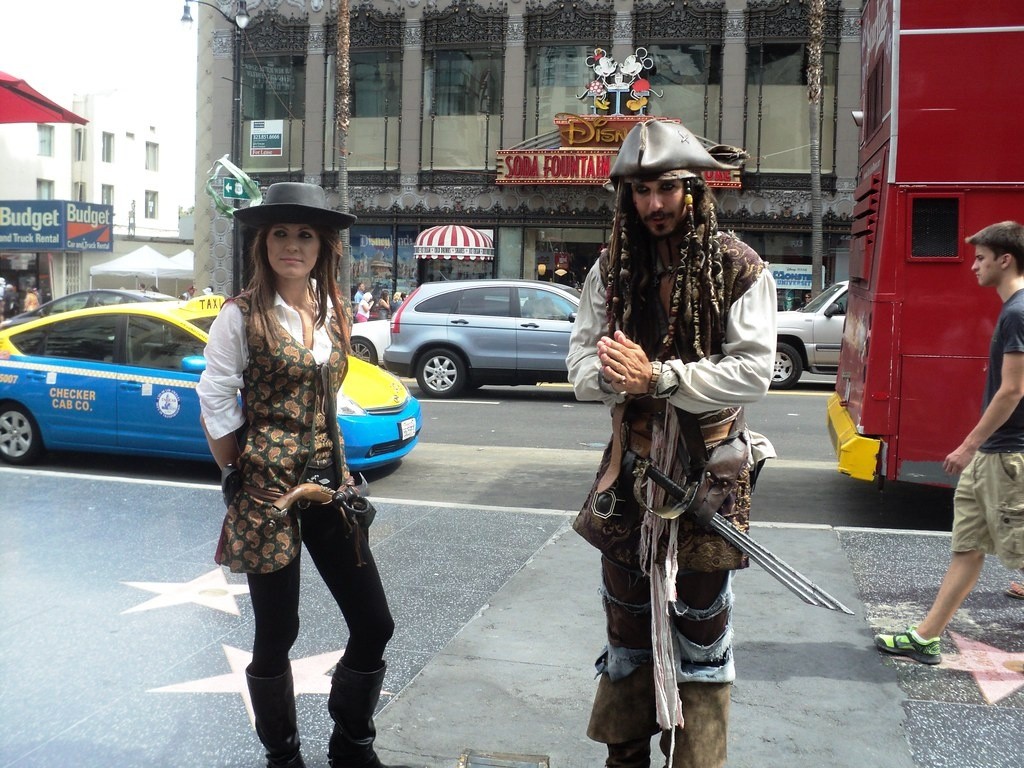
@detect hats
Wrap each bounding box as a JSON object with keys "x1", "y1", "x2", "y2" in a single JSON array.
[
  {"x1": 608, "y1": 119, "x2": 750, "y2": 183},
  {"x1": 234, "y1": 182, "x2": 359, "y2": 230}
]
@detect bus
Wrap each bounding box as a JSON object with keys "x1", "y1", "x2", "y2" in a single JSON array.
[{"x1": 826, "y1": 1, "x2": 1024, "y2": 488}]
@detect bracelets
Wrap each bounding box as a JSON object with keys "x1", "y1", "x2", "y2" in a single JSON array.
[{"x1": 647, "y1": 362, "x2": 661, "y2": 395}]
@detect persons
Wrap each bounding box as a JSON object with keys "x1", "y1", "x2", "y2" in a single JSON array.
[
  {"x1": 194, "y1": 182, "x2": 410, "y2": 768},
  {"x1": 0, "y1": 277, "x2": 45, "y2": 317},
  {"x1": 799, "y1": 293, "x2": 812, "y2": 311},
  {"x1": 565, "y1": 118, "x2": 777, "y2": 768},
  {"x1": 119, "y1": 284, "x2": 215, "y2": 300},
  {"x1": 874, "y1": 221, "x2": 1024, "y2": 665},
  {"x1": 354, "y1": 282, "x2": 412, "y2": 322}
]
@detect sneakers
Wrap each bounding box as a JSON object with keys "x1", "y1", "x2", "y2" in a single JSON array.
[{"x1": 874, "y1": 623, "x2": 941, "y2": 665}]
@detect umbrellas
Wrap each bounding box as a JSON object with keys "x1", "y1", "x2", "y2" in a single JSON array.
[{"x1": 0, "y1": 70, "x2": 89, "y2": 125}]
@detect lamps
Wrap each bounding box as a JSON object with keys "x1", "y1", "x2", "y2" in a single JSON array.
[
  {"x1": 555, "y1": 227, "x2": 568, "y2": 276},
  {"x1": 537, "y1": 256, "x2": 547, "y2": 275}
]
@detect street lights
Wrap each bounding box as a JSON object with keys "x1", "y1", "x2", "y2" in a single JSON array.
[{"x1": 181, "y1": 0, "x2": 250, "y2": 298}]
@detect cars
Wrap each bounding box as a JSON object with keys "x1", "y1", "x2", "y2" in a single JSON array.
[
  {"x1": 0, "y1": 295, "x2": 422, "y2": 473},
  {"x1": 0, "y1": 289, "x2": 184, "y2": 329},
  {"x1": 349, "y1": 297, "x2": 528, "y2": 366}
]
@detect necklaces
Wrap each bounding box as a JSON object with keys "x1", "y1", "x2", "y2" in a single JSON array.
[{"x1": 661, "y1": 260, "x2": 683, "y2": 280}]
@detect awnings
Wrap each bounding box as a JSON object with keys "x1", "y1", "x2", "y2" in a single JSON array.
[{"x1": 413, "y1": 225, "x2": 495, "y2": 261}]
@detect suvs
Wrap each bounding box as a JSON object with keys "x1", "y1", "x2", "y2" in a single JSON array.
[
  {"x1": 768, "y1": 278, "x2": 852, "y2": 390},
  {"x1": 381, "y1": 277, "x2": 583, "y2": 400}
]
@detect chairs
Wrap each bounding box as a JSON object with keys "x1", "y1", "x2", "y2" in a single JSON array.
[{"x1": 523, "y1": 300, "x2": 534, "y2": 317}]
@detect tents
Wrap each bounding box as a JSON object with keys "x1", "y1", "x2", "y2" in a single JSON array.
[{"x1": 89, "y1": 245, "x2": 195, "y2": 290}]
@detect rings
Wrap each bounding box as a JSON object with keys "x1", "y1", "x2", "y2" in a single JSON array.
[{"x1": 619, "y1": 375, "x2": 628, "y2": 384}]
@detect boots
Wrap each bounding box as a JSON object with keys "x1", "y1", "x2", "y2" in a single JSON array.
[
  {"x1": 328, "y1": 659, "x2": 405, "y2": 768},
  {"x1": 247, "y1": 658, "x2": 304, "y2": 768}
]
